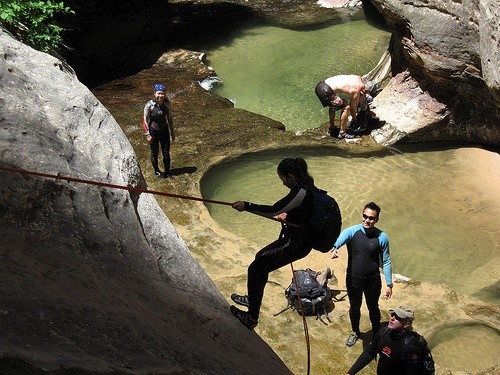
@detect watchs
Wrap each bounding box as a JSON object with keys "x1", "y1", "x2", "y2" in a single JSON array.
[{"x1": 387, "y1": 283, "x2": 394, "y2": 288}]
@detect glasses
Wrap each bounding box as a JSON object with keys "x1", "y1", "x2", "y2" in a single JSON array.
[
  {"x1": 390, "y1": 312, "x2": 402, "y2": 321},
  {"x1": 363, "y1": 215, "x2": 375, "y2": 220}
]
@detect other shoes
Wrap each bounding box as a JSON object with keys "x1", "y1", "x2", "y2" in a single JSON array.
[
  {"x1": 327, "y1": 126, "x2": 340, "y2": 136},
  {"x1": 347, "y1": 330, "x2": 360, "y2": 346},
  {"x1": 338, "y1": 132, "x2": 353, "y2": 139}
]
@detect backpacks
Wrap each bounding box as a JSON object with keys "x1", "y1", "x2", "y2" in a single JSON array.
[
  {"x1": 351, "y1": 106, "x2": 371, "y2": 135},
  {"x1": 381, "y1": 327, "x2": 435, "y2": 375},
  {"x1": 285, "y1": 269, "x2": 332, "y2": 315},
  {"x1": 304, "y1": 187, "x2": 342, "y2": 254}
]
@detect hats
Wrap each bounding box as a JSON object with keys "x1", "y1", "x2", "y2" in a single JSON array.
[
  {"x1": 389, "y1": 306, "x2": 414, "y2": 321},
  {"x1": 154, "y1": 85, "x2": 166, "y2": 92}
]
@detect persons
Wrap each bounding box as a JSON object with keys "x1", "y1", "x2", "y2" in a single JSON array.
[
  {"x1": 315, "y1": 75, "x2": 377, "y2": 138},
  {"x1": 230, "y1": 157, "x2": 318, "y2": 331},
  {"x1": 346, "y1": 306, "x2": 435, "y2": 375},
  {"x1": 143, "y1": 84, "x2": 175, "y2": 177},
  {"x1": 331, "y1": 202, "x2": 394, "y2": 348}
]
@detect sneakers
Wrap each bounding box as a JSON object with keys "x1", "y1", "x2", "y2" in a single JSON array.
[
  {"x1": 231, "y1": 293, "x2": 249, "y2": 308},
  {"x1": 230, "y1": 305, "x2": 257, "y2": 330}
]
